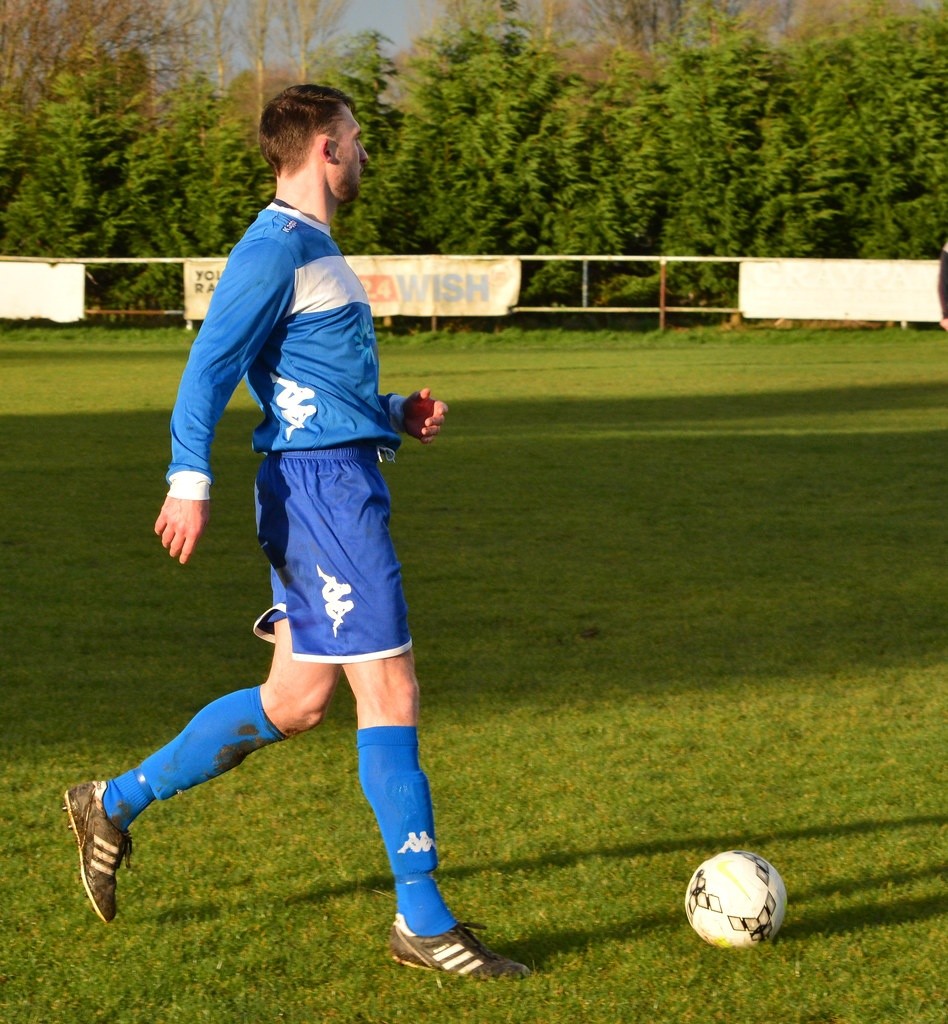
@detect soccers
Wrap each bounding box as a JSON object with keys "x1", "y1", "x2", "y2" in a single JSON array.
[{"x1": 684, "y1": 849, "x2": 788, "y2": 948}]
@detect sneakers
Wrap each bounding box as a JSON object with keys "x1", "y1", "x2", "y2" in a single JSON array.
[
  {"x1": 62, "y1": 779, "x2": 132, "y2": 924},
  {"x1": 389, "y1": 911, "x2": 531, "y2": 984}
]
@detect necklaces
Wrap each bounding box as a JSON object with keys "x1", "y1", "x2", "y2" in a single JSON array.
[{"x1": 272, "y1": 198, "x2": 293, "y2": 210}]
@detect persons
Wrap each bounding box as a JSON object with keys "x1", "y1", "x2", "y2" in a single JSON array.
[{"x1": 63, "y1": 84, "x2": 530, "y2": 982}]
